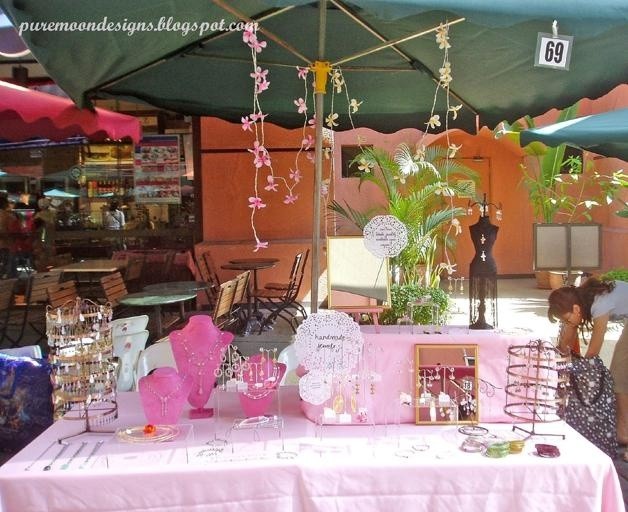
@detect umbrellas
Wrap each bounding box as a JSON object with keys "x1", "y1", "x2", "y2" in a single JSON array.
[
  {"x1": 0, "y1": 0, "x2": 628, "y2": 317},
  {"x1": 519, "y1": 111, "x2": 628, "y2": 161}
]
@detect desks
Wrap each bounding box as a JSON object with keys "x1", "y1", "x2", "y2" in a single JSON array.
[{"x1": 0, "y1": 386, "x2": 615, "y2": 512}]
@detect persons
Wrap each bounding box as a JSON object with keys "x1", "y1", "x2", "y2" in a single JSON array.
[
  {"x1": 547, "y1": 278, "x2": 628, "y2": 447},
  {"x1": 467, "y1": 214, "x2": 499, "y2": 327},
  {"x1": 101, "y1": 200, "x2": 125, "y2": 231},
  {"x1": 0, "y1": 189, "x2": 74, "y2": 280}
]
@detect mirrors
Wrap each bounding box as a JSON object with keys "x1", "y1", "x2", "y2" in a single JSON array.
[{"x1": 326, "y1": 233, "x2": 391, "y2": 310}]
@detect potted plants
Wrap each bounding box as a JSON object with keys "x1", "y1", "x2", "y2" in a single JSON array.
[{"x1": 494, "y1": 101, "x2": 628, "y2": 290}]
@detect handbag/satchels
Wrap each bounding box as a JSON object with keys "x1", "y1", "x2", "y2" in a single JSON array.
[{"x1": 556, "y1": 349, "x2": 619, "y2": 460}]
